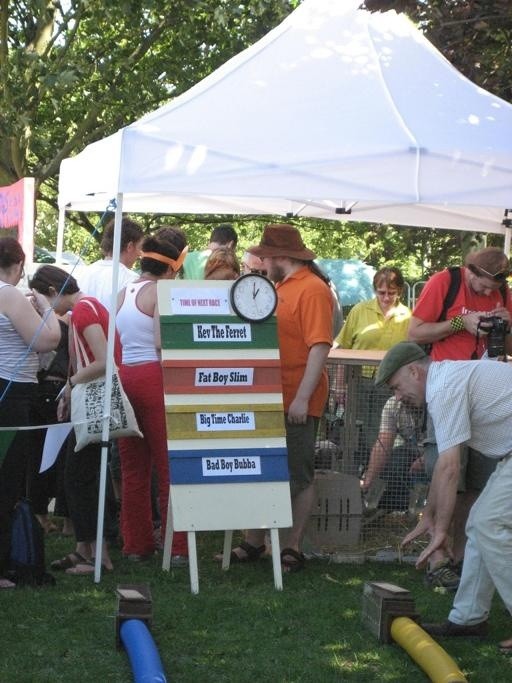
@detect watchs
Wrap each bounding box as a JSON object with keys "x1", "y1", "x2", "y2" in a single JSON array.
[{"x1": 68, "y1": 377, "x2": 74, "y2": 389}]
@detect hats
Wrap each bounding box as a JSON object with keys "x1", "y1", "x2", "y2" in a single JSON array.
[
  {"x1": 245, "y1": 225, "x2": 317, "y2": 260},
  {"x1": 375, "y1": 342, "x2": 425, "y2": 387},
  {"x1": 466, "y1": 246, "x2": 508, "y2": 279}
]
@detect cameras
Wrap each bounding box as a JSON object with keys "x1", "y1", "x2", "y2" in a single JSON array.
[{"x1": 470, "y1": 315, "x2": 510, "y2": 361}]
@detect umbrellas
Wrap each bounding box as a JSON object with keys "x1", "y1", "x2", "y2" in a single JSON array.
[{"x1": 312, "y1": 259, "x2": 379, "y2": 306}]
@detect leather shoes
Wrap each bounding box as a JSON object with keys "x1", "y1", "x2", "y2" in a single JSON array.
[
  {"x1": 421, "y1": 619, "x2": 491, "y2": 637},
  {"x1": 498, "y1": 638, "x2": 512, "y2": 651}
]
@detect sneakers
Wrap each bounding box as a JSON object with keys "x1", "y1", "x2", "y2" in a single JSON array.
[
  {"x1": 128, "y1": 550, "x2": 159, "y2": 562},
  {"x1": 426, "y1": 563, "x2": 461, "y2": 592},
  {"x1": 171, "y1": 554, "x2": 190, "y2": 567}
]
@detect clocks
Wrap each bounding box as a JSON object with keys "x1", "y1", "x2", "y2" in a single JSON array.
[{"x1": 229, "y1": 272, "x2": 277, "y2": 324}]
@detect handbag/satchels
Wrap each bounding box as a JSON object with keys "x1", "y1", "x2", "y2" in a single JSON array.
[{"x1": 69, "y1": 301, "x2": 144, "y2": 452}]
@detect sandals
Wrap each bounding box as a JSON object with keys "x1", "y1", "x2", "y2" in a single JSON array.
[
  {"x1": 279, "y1": 549, "x2": 305, "y2": 574},
  {"x1": 65, "y1": 559, "x2": 112, "y2": 575},
  {"x1": 50, "y1": 554, "x2": 87, "y2": 570},
  {"x1": 213, "y1": 541, "x2": 267, "y2": 564}
]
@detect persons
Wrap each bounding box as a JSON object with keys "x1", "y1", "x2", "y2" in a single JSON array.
[
  {"x1": 213, "y1": 225, "x2": 335, "y2": 575},
  {"x1": 375, "y1": 342, "x2": 512, "y2": 648},
  {"x1": 205, "y1": 247, "x2": 240, "y2": 279},
  {"x1": 0, "y1": 238, "x2": 60, "y2": 588},
  {"x1": 74, "y1": 218, "x2": 143, "y2": 319},
  {"x1": 27, "y1": 265, "x2": 123, "y2": 574},
  {"x1": 358, "y1": 396, "x2": 438, "y2": 514},
  {"x1": 332, "y1": 267, "x2": 412, "y2": 453},
  {"x1": 178, "y1": 224, "x2": 237, "y2": 279},
  {"x1": 115, "y1": 226, "x2": 189, "y2": 566},
  {"x1": 409, "y1": 247, "x2": 512, "y2": 590}
]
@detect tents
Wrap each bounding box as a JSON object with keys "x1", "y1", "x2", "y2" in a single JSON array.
[{"x1": 57, "y1": 0, "x2": 512, "y2": 582}]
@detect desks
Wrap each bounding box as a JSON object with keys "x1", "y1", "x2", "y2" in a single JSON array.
[{"x1": 326, "y1": 348, "x2": 389, "y2": 366}]
